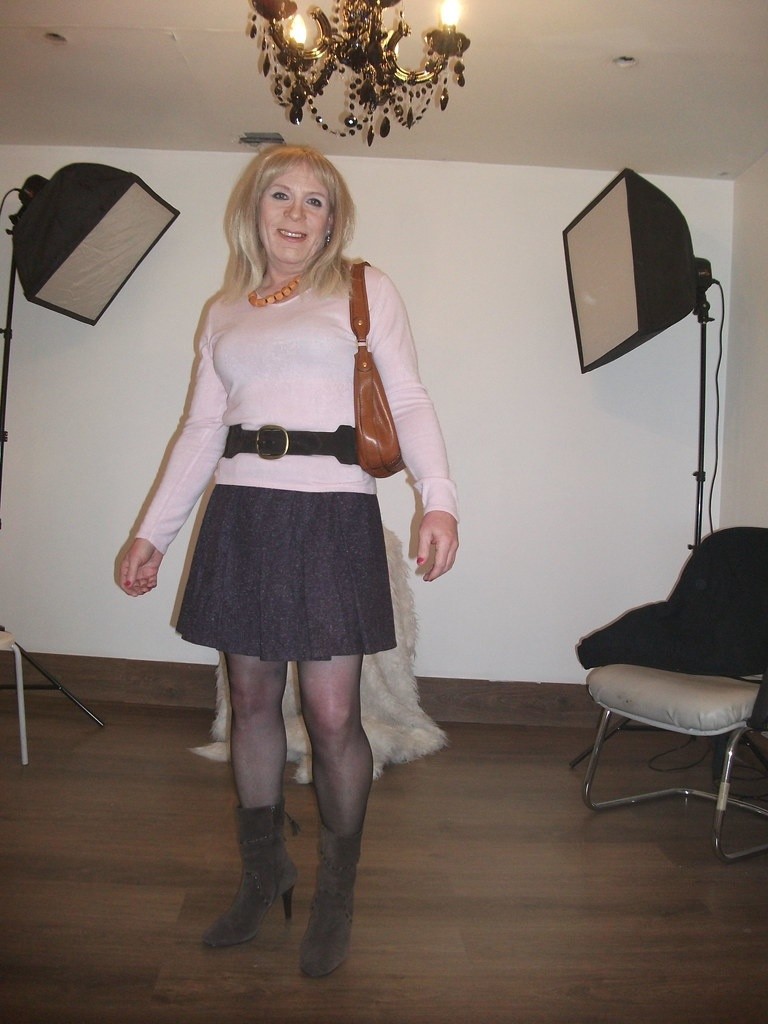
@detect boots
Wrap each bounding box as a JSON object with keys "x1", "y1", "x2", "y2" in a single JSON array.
[
  {"x1": 202, "y1": 797, "x2": 297, "y2": 944},
  {"x1": 300, "y1": 821, "x2": 362, "y2": 977}
]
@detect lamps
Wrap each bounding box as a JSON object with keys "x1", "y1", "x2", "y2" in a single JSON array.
[{"x1": 248, "y1": 0, "x2": 471, "y2": 147}]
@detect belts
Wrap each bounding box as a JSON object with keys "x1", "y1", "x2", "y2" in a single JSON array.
[{"x1": 222, "y1": 425, "x2": 361, "y2": 465}]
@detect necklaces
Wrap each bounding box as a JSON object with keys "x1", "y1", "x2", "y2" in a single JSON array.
[{"x1": 248, "y1": 276, "x2": 302, "y2": 307}]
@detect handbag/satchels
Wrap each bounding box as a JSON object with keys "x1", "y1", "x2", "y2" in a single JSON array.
[{"x1": 349, "y1": 261, "x2": 406, "y2": 478}]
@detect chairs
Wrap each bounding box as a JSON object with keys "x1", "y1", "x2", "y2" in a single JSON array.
[{"x1": 583, "y1": 525, "x2": 768, "y2": 867}]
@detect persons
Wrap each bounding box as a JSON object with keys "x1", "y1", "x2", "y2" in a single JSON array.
[{"x1": 118, "y1": 145, "x2": 459, "y2": 978}]
